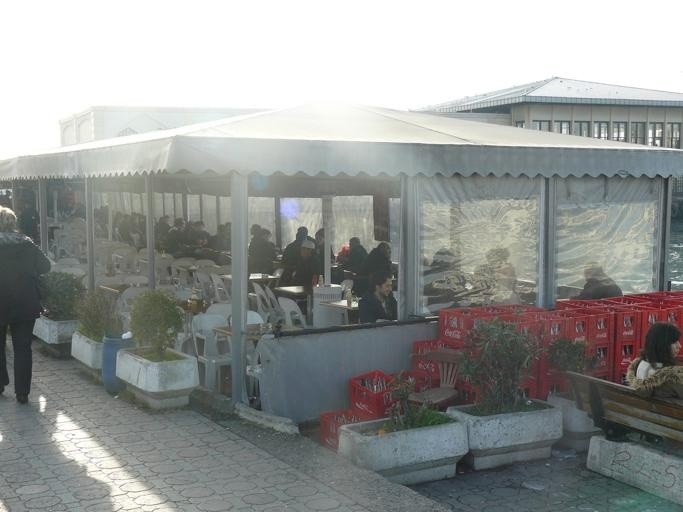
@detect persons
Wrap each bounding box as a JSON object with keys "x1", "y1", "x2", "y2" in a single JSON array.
[
  {"x1": 569, "y1": 262, "x2": 622, "y2": 300},
  {"x1": 0, "y1": 207, "x2": 51, "y2": 403},
  {"x1": 18, "y1": 201, "x2": 519, "y2": 323},
  {"x1": 627, "y1": 323, "x2": 683, "y2": 400}
]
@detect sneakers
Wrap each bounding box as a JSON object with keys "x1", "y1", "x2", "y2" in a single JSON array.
[{"x1": 14, "y1": 391, "x2": 30, "y2": 403}]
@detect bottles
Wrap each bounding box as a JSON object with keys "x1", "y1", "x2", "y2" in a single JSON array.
[
  {"x1": 346, "y1": 289, "x2": 353, "y2": 306},
  {"x1": 186, "y1": 290, "x2": 209, "y2": 315},
  {"x1": 311, "y1": 275, "x2": 323, "y2": 286},
  {"x1": 332, "y1": 340, "x2": 477, "y2": 438},
  {"x1": 452, "y1": 293, "x2": 683, "y2": 399}
]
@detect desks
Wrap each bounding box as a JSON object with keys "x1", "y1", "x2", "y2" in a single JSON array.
[{"x1": 319, "y1": 290, "x2": 428, "y2": 324}]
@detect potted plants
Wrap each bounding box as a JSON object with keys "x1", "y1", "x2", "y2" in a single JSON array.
[{"x1": 338, "y1": 374, "x2": 468, "y2": 487}]
